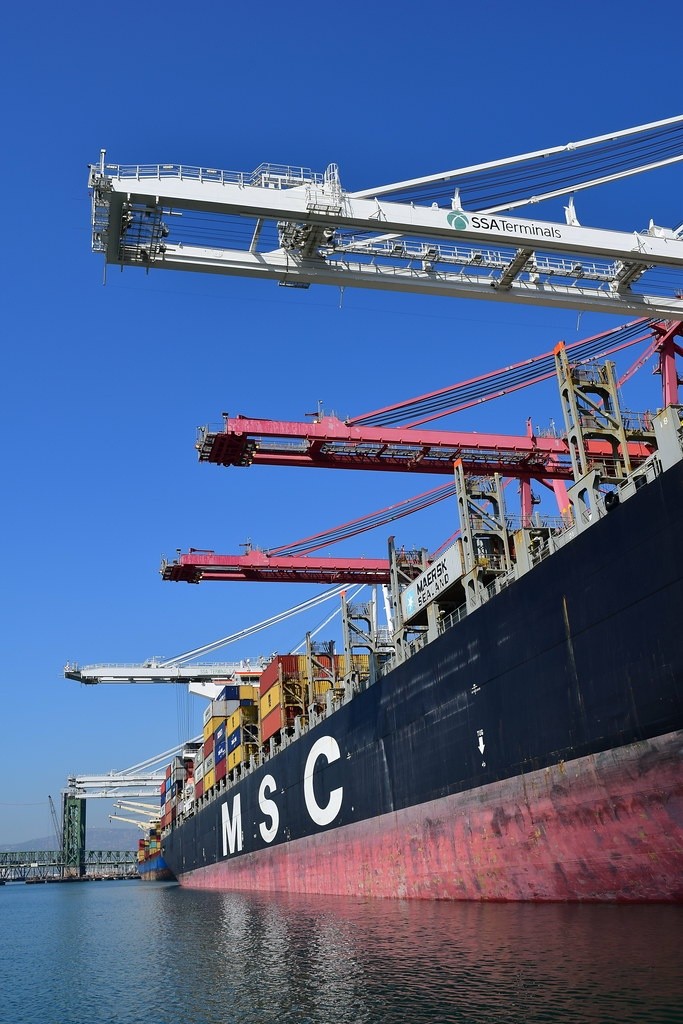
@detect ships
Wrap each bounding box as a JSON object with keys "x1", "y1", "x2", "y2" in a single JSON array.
[{"x1": 55, "y1": 109, "x2": 681, "y2": 901}]
